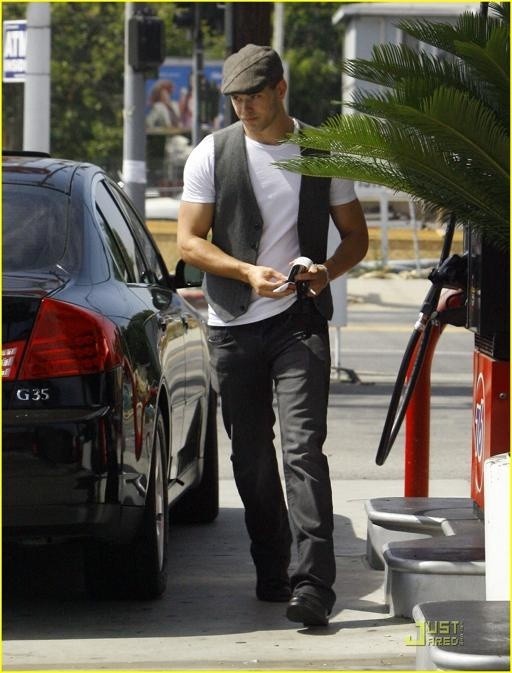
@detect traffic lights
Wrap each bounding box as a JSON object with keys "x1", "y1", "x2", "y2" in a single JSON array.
[{"x1": 130, "y1": 13, "x2": 166, "y2": 80}]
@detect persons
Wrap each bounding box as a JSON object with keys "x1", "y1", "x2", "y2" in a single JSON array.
[
  {"x1": 145, "y1": 78, "x2": 179, "y2": 129},
  {"x1": 180, "y1": 73, "x2": 209, "y2": 128},
  {"x1": 175, "y1": 42, "x2": 372, "y2": 627}
]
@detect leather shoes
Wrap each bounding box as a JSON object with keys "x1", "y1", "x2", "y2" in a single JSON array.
[
  {"x1": 285, "y1": 596, "x2": 329, "y2": 628},
  {"x1": 255, "y1": 573, "x2": 292, "y2": 603}
]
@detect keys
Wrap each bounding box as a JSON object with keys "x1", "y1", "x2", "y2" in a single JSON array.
[{"x1": 300, "y1": 279, "x2": 317, "y2": 296}]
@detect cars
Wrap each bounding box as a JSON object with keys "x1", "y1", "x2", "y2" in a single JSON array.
[{"x1": 1, "y1": 149, "x2": 218, "y2": 601}]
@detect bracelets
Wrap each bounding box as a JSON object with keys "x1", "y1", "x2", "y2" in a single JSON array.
[{"x1": 318, "y1": 262, "x2": 332, "y2": 290}]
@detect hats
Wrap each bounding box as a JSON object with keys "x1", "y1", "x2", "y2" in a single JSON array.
[{"x1": 221, "y1": 44, "x2": 283, "y2": 96}]
